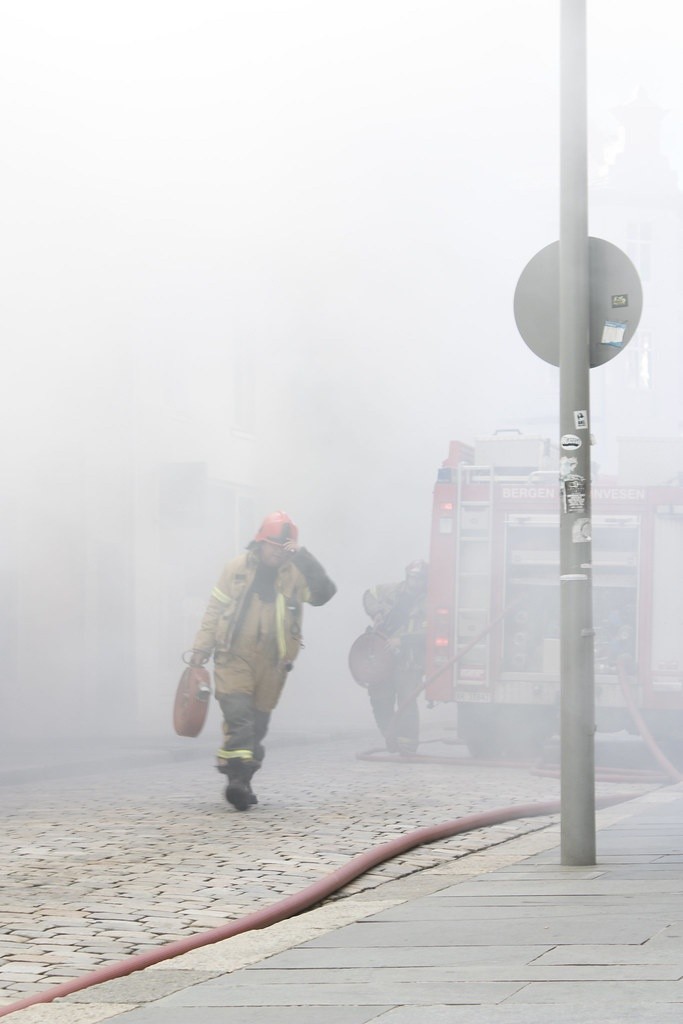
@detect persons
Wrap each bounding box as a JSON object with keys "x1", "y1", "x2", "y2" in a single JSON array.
[
  {"x1": 363, "y1": 558, "x2": 427, "y2": 756},
  {"x1": 190, "y1": 511, "x2": 337, "y2": 810}
]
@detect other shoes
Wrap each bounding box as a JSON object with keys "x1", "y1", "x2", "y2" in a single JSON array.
[
  {"x1": 226, "y1": 778, "x2": 259, "y2": 810},
  {"x1": 386, "y1": 737, "x2": 399, "y2": 754}
]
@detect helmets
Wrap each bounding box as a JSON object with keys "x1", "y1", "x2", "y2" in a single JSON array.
[
  {"x1": 255, "y1": 512, "x2": 298, "y2": 550},
  {"x1": 406, "y1": 559, "x2": 427, "y2": 582}
]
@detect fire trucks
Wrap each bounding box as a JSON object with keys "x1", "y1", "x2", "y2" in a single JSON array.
[{"x1": 418, "y1": 424, "x2": 683, "y2": 773}]
